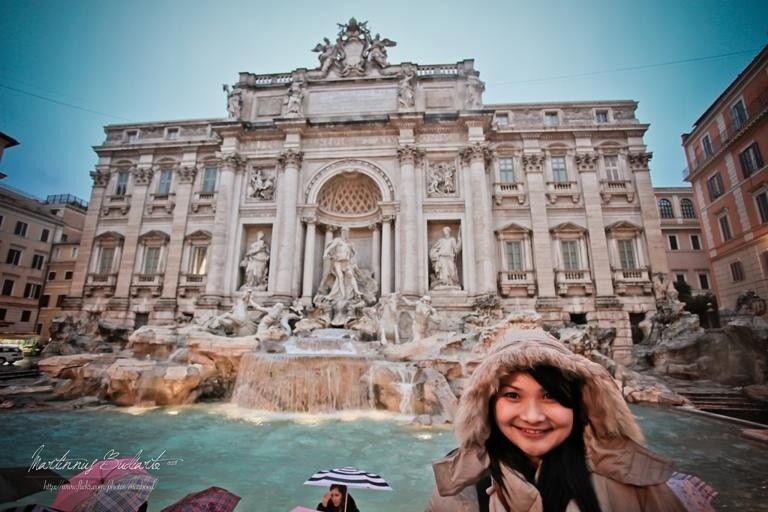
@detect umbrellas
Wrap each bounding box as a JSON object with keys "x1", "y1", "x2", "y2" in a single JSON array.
[
  {"x1": 672, "y1": 471, "x2": 719, "y2": 504},
  {"x1": 303, "y1": 465, "x2": 392, "y2": 512},
  {"x1": 0, "y1": 457, "x2": 242, "y2": 512},
  {"x1": 665, "y1": 479, "x2": 719, "y2": 512}
]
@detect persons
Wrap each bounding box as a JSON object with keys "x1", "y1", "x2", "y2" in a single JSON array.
[
  {"x1": 247, "y1": 229, "x2": 270, "y2": 286},
  {"x1": 259, "y1": 175, "x2": 275, "y2": 199},
  {"x1": 315, "y1": 32, "x2": 388, "y2": 70},
  {"x1": 316, "y1": 484, "x2": 360, "y2": 512},
  {"x1": 323, "y1": 227, "x2": 364, "y2": 299},
  {"x1": 430, "y1": 167, "x2": 442, "y2": 193},
  {"x1": 252, "y1": 170, "x2": 267, "y2": 199},
  {"x1": 204, "y1": 289, "x2": 441, "y2": 346},
  {"x1": 397, "y1": 72, "x2": 415, "y2": 108},
  {"x1": 423, "y1": 325, "x2": 688, "y2": 511},
  {"x1": 433, "y1": 225, "x2": 462, "y2": 286},
  {"x1": 465, "y1": 69, "x2": 482, "y2": 104},
  {"x1": 443, "y1": 165, "x2": 453, "y2": 193},
  {"x1": 282, "y1": 76, "x2": 306, "y2": 114},
  {"x1": 223, "y1": 81, "x2": 243, "y2": 120}
]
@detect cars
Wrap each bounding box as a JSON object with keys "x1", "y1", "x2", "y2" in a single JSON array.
[
  {"x1": 0, "y1": 345, "x2": 23, "y2": 364},
  {"x1": 23, "y1": 345, "x2": 41, "y2": 356}
]
